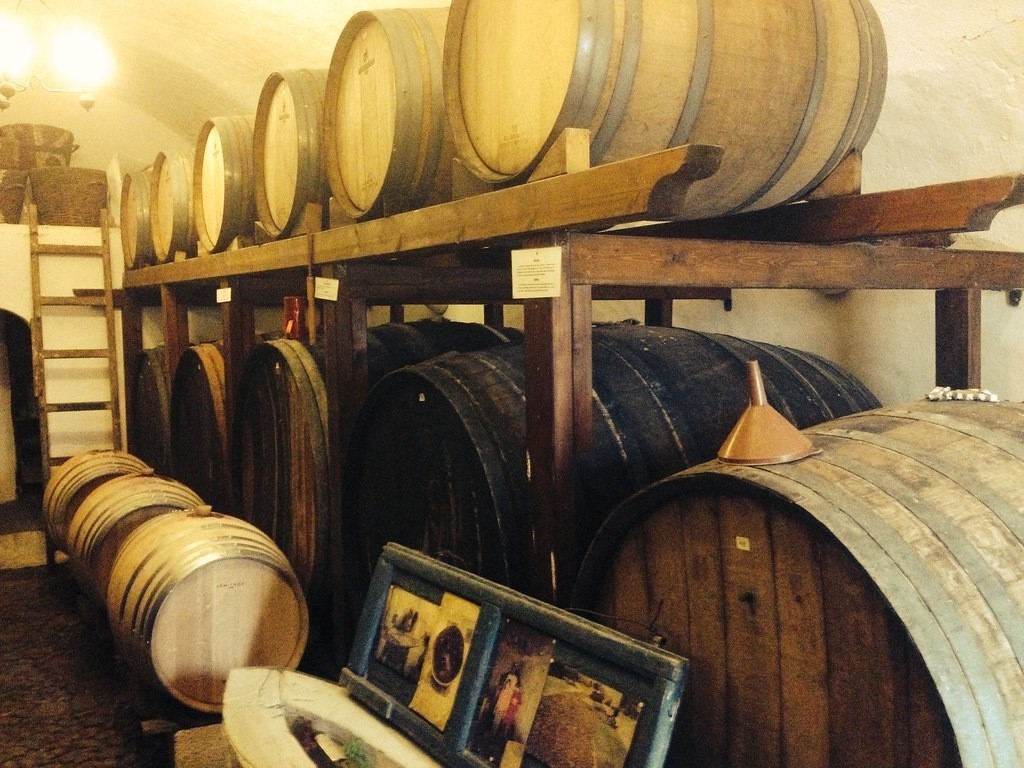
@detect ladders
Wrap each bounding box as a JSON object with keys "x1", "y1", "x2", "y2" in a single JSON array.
[{"x1": 28, "y1": 204, "x2": 128, "y2": 569}]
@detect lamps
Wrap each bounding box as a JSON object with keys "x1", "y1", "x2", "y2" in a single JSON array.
[{"x1": 0, "y1": 0, "x2": 118, "y2": 113}]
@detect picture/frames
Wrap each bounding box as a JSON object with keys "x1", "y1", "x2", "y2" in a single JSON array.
[{"x1": 341, "y1": 543, "x2": 687, "y2": 768}]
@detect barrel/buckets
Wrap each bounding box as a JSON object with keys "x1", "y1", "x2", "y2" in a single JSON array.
[{"x1": 0, "y1": 0, "x2": 1024, "y2": 768}]
